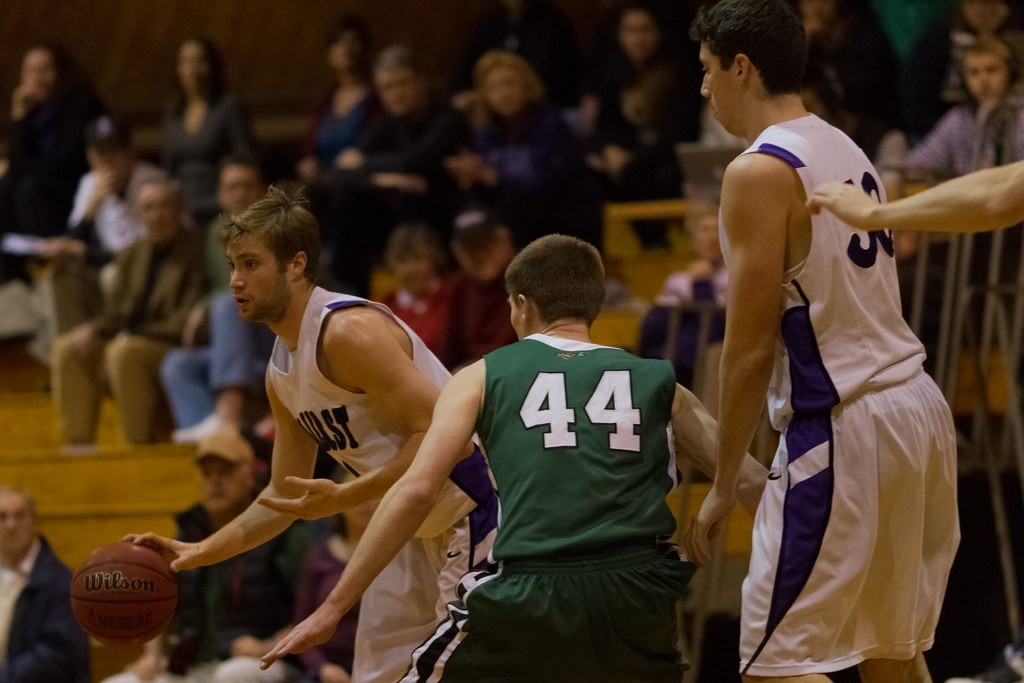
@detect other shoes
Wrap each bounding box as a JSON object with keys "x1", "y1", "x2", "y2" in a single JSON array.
[{"x1": 169, "y1": 414, "x2": 238, "y2": 453}]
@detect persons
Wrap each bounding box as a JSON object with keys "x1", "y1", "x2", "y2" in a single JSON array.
[{"x1": 0, "y1": 0, "x2": 1024, "y2": 683}]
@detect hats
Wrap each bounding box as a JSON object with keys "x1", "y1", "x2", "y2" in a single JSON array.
[
  {"x1": 85, "y1": 114, "x2": 131, "y2": 145},
  {"x1": 192, "y1": 427, "x2": 255, "y2": 464}
]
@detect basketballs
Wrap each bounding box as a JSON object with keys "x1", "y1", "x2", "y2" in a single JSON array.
[{"x1": 70, "y1": 541, "x2": 178, "y2": 648}]
